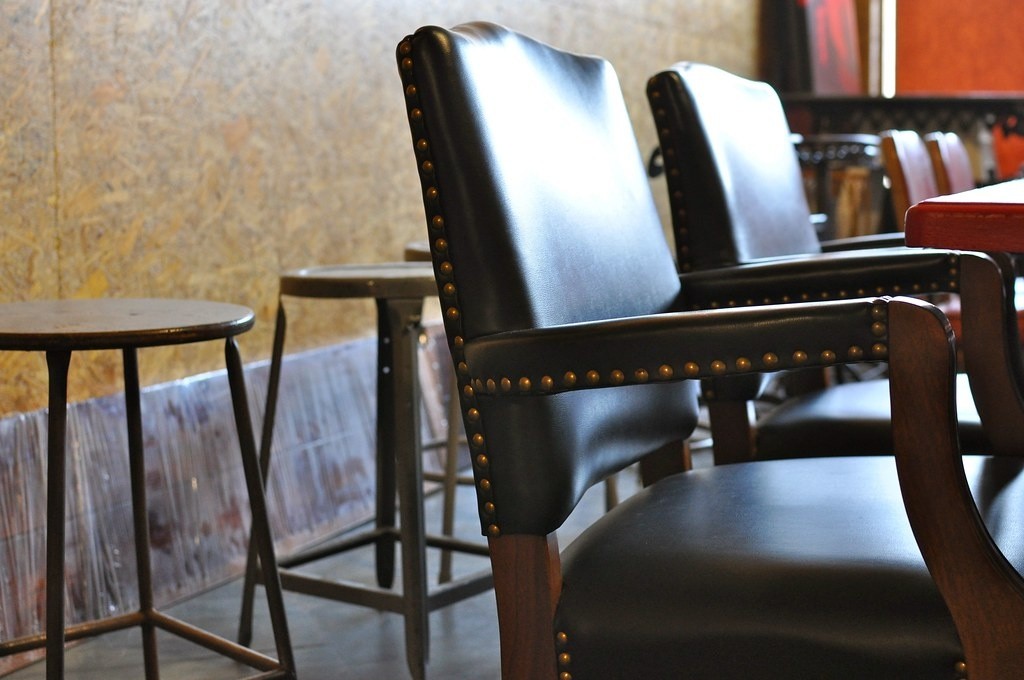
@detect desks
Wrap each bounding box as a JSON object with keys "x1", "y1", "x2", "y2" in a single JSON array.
[{"x1": 904, "y1": 176, "x2": 1023, "y2": 250}]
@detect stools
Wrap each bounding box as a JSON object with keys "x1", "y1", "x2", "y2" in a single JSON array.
[
  {"x1": 401, "y1": 239, "x2": 615, "y2": 584},
  {"x1": 1, "y1": 300, "x2": 300, "y2": 680},
  {"x1": 236, "y1": 260, "x2": 498, "y2": 680}
]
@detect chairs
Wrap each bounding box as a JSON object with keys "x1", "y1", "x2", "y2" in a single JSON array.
[
  {"x1": 923, "y1": 132, "x2": 979, "y2": 196},
  {"x1": 644, "y1": 62, "x2": 1024, "y2": 464},
  {"x1": 395, "y1": 21, "x2": 1024, "y2": 680},
  {"x1": 881, "y1": 128, "x2": 942, "y2": 231}
]
